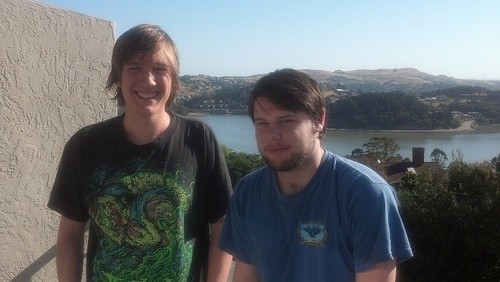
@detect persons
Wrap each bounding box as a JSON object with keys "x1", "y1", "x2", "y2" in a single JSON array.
[
  {"x1": 212, "y1": 68, "x2": 415, "y2": 281},
  {"x1": 46, "y1": 21, "x2": 235, "y2": 282}
]
